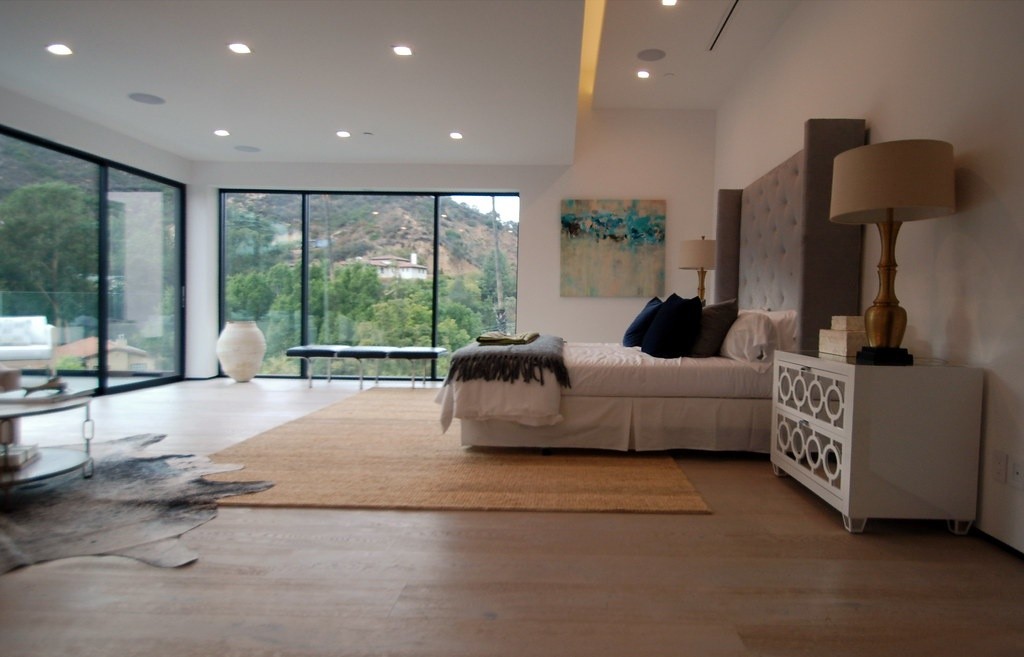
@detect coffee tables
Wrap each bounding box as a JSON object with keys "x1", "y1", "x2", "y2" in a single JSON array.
[{"x1": 0, "y1": 387, "x2": 96, "y2": 486}]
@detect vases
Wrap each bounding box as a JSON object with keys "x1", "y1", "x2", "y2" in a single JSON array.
[{"x1": 215, "y1": 320, "x2": 266, "y2": 381}]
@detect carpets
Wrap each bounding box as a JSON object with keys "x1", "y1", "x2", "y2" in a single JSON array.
[
  {"x1": 0, "y1": 433, "x2": 277, "y2": 575},
  {"x1": 202, "y1": 386, "x2": 717, "y2": 515}
]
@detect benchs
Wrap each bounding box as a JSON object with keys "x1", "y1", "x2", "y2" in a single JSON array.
[{"x1": 286, "y1": 345, "x2": 448, "y2": 392}]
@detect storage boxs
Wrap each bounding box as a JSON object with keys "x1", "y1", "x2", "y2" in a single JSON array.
[
  {"x1": 831, "y1": 315, "x2": 866, "y2": 330},
  {"x1": 819, "y1": 328, "x2": 870, "y2": 356}
]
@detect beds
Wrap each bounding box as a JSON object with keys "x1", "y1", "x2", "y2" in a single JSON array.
[{"x1": 434, "y1": 118, "x2": 866, "y2": 452}]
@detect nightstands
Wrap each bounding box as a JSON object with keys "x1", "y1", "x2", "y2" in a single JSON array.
[{"x1": 770, "y1": 349, "x2": 983, "y2": 533}]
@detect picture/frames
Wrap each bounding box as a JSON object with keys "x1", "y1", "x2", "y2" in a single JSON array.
[{"x1": 559, "y1": 198, "x2": 665, "y2": 296}]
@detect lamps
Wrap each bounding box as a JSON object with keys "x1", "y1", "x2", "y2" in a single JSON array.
[
  {"x1": 677, "y1": 235, "x2": 718, "y2": 306},
  {"x1": 828, "y1": 138, "x2": 955, "y2": 366}
]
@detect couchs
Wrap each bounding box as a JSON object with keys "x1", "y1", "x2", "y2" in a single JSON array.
[{"x1": 0, "y1": 315, "x2": 59, "y2": 377}]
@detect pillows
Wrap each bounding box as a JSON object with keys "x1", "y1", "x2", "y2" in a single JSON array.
[{"x1": 622, "y1": 293, "x2": 797, "y2": 373}]
